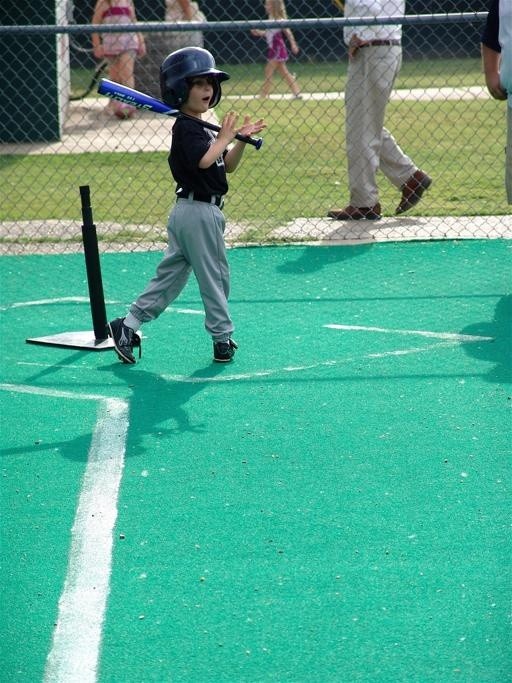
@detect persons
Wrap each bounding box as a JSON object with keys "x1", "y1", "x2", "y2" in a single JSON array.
[
  {"x1": 103, "y1": 44, "x2": 267, "y2": 366},
  {"x1": 326, "y1": 0, "x2": 434, "y2": 221},
  {"x1": 92, "y1": 0, "x2": 147, "y2": 119},
  {"x1": 162, "y1": 0, "x2": 209, "y2": 53},
  {"x1": 479, "y1": 0, "x2": 512, "y2": 207},
  {"x1": 248, "y1": 1, "x2": 304, "y2": 100}
]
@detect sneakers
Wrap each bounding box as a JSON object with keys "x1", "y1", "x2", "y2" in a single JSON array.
[
  {"x1": 115, "y1": 104, "x2": 135, "y2": 119},
  {"x1": 104, "y1": 316, "x2": 141, "y2": 363},
  {"x1": 213, "y1": 338, "x2": 238, "y2": 361}
]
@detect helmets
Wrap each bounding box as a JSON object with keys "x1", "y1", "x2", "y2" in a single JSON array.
[{"x1": 159, "y1": 46, "x2": 230, "y2": 108}]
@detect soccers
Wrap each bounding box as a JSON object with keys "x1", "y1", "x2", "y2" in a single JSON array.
[{"x1": 110, "y1": 98, "x2": 136, "y2": 118}]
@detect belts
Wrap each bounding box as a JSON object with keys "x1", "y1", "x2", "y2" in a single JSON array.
[
  {"x1": 177, "y1": 190, "x2": 225, "y2": 210},
  {"x1": 360, "y1": 38, "x2": 401, "y2": 47}
]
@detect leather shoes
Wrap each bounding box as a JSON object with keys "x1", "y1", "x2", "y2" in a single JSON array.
[
  {"x1": 396, "y1": 168, "x2": 433, "y2": 213},
  {"x1": 327, "y1": 202, "x2": 382, "y2": 220}
]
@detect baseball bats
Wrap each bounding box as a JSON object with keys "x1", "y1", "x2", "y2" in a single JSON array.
[{"x1": 97, "y1": 79, "x2": 263, "y2": 148}]
